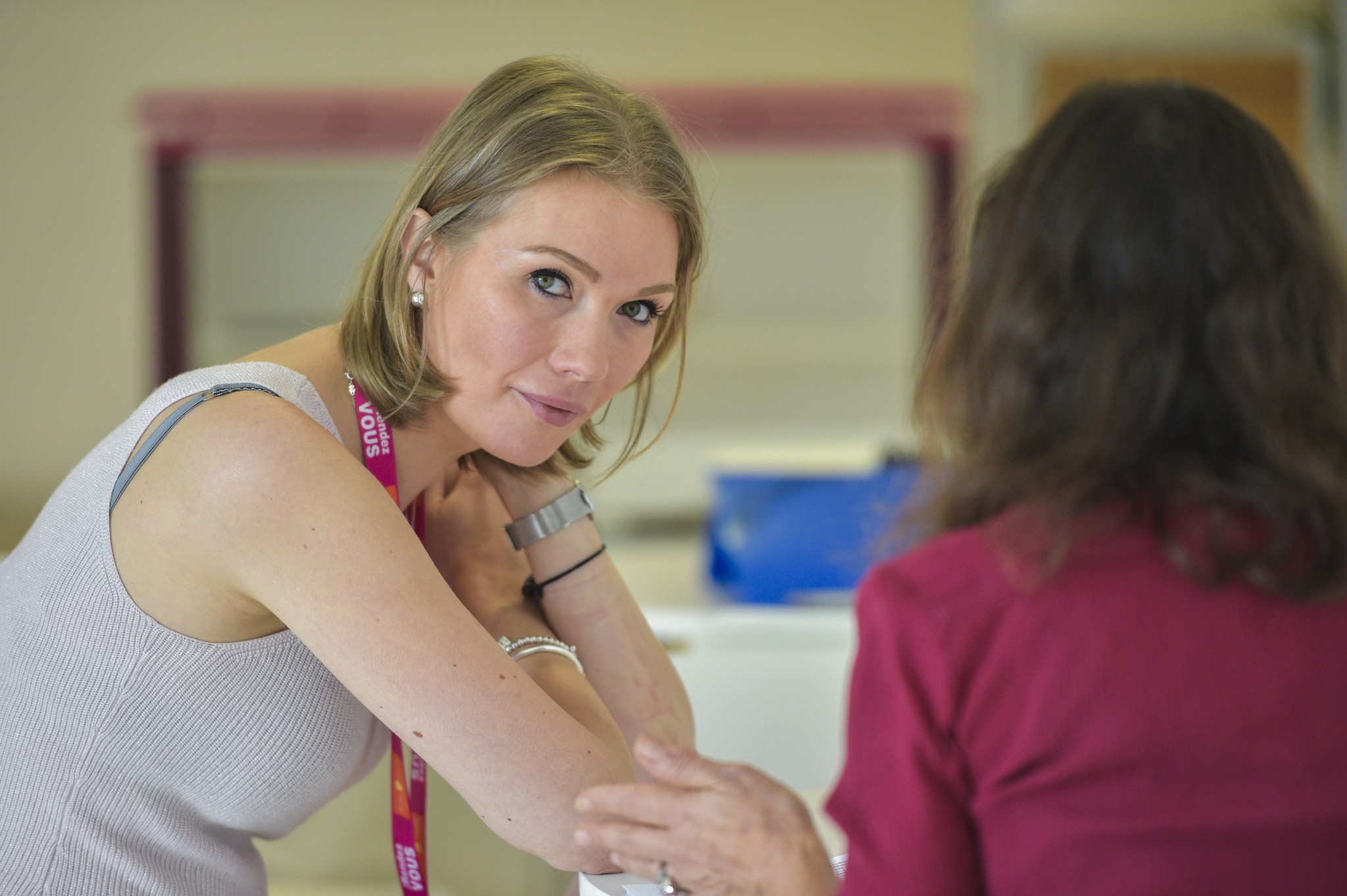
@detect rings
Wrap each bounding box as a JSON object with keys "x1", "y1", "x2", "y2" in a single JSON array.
[{"x1": 655, "y1": 857, "x2": 677, "y2": 895}]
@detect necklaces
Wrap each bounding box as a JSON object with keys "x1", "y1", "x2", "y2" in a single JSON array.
[{"x1": 345, "y1": 371, "x2": 356, "y2": 408}]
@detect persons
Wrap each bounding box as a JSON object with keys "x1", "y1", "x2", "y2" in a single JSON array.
[
  {"x1": 7, "y1": 62, "x2": 705, "y2": 893},
  {"x1": 572, "y1": 81, "x2": 1347, "y2": 896}
]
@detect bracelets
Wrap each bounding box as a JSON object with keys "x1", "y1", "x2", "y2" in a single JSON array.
[
  {"x1": 499, "y1": 634, "x2": 578, "y2": 656},
  {"x1": 505, "y1": 480, "x2": 594, "y2": 552},
  {"x1": 520, "y1": 543, "x2": 605, "y2": 604},
  {"x1": 511, "y1": 643, "x2": 583, "y2": 675}
]
